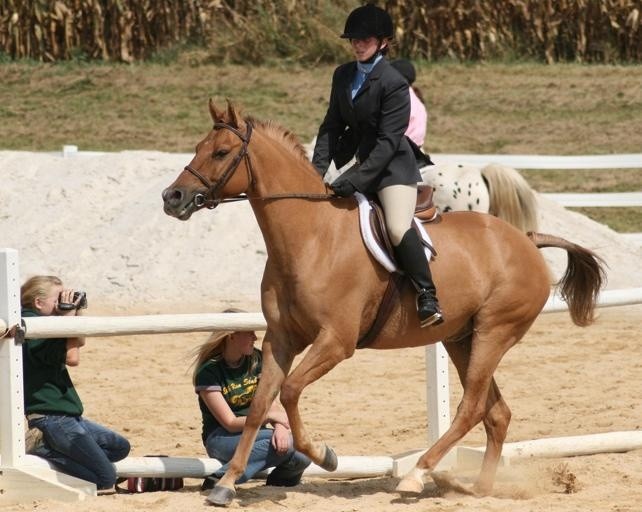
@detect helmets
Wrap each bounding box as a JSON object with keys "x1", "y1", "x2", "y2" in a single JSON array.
[
  {"x1": 390, "y1": 59, "x2": 417, "y2": 85},
  {"x1": 340, "y1": 2, "x2": 393, "y2": 41}
]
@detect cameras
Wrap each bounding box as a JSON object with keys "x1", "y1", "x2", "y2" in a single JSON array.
[{"x1": 56, "y1": 291, "x2": 88, "y2": 312}]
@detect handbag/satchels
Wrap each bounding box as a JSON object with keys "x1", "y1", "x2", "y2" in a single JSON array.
[{"x1": 126, "y1": 454, "x2": 184, "y2": 493}]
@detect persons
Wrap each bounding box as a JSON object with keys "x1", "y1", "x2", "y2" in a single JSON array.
[
  {"x1": 310, "y1": 3, "x2": 444, "y2": 327},
  {"x1": 389, "y1": 60, "x2": 429, "y2": 155},
  {"x1": 19, "y1": 274, "x2": 131, "y2": 490},
  {"x1": 191, "y1": 307, "x2": 313, "y2": 491}
]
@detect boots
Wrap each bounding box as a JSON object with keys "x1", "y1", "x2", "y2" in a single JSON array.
[{"x1": 396, "y1": 228, "x2": 439, "y2": 319}]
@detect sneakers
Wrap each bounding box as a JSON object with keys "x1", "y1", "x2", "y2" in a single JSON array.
[
  {"x1": 26, "y1": 427, "x2": 43, "y2": 453},
  {"x1": 200, "y1": 474, "x2": 219, "y2": 491}
]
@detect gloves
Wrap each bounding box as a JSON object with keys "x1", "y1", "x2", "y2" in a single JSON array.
[{"x1": 327, "y1": 178, "x2": 355, "y2": 198}]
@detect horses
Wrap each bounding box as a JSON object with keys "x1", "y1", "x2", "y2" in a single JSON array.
[
  {"x1": 302, "y1": 135, "x2": 541, "y2": 247},
  {"x1": 161, "y1": 98, "x2": 611, "y2": 506}
]
